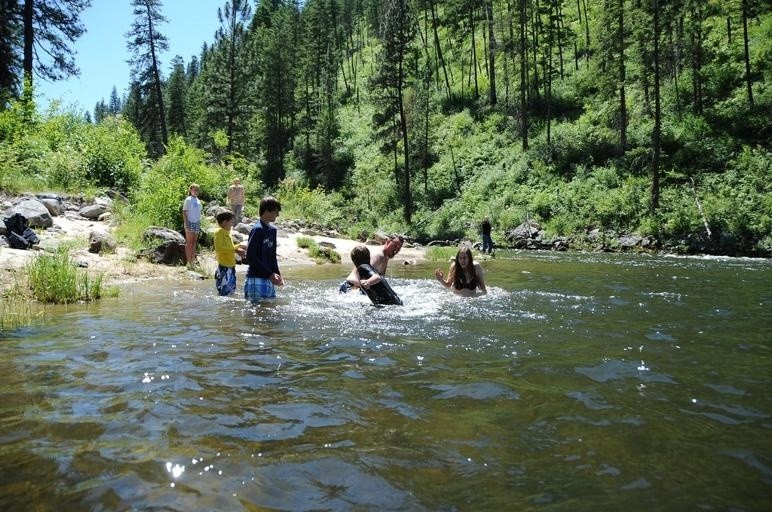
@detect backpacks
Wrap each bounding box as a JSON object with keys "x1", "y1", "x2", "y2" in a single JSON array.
[{"x1": 3, "y1": 212, "x2": 39, "y2": 249}]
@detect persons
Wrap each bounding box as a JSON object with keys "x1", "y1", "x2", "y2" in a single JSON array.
[
  {"x1": 350, "y1": 245, "x2": 404, "y2": 310},
  {"x1": 244, "y1": 196, "x2": 285, "y2": 300},
  {"x1": 226, "y1": 177, "x2": 245, "y2": 227},
  {"x1": 182, "y1": 182, "x2": 203, "y2": 269},
  {"x1": 481, "y1": 215, "x2": 494, "y2": 255},
  {"x1": 212, "y1": 211, "x2": 247, "y2": 296},
  {"x1": 339, "y1": 234, "x2": 404, "y2": 296},
  {"x1": 435, "y1": 246, "x2": 489, "y2": 300}
]
